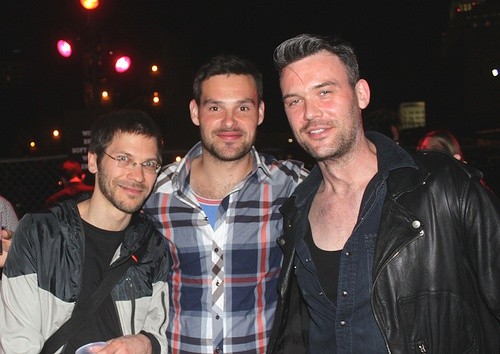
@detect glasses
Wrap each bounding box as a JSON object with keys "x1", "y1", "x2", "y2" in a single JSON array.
[{"x1": 102, "y1": 150, "x2": 163, "y2": 174}]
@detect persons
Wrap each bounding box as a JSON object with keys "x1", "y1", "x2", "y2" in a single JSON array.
[
  {"x1": 415, "y1": 129, "x2": 465, "y2": 161},
  {"x1": 0, "y1": 56, "x2": 312, "y2": 354},
  {"x1": 266, "y1": 33, "x2": 500, "y2": 354},
  {"x1": 0, "y1": 195, "x2": 18, "y2": 234},
  {"x1": 46, "y1": 160, "x2": 95, "y2": 212},
  {"x1": 0, "y1": 110, "x2": 171, "y2": 354}
]
